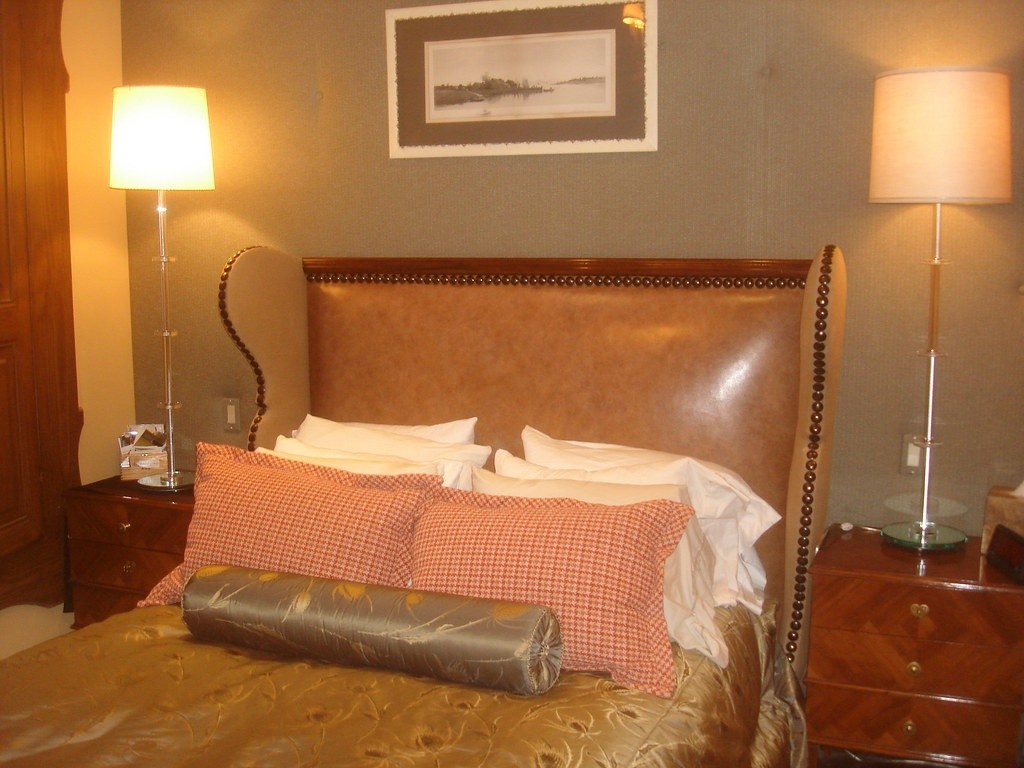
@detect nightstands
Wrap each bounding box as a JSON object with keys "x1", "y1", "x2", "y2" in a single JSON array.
[
  {"x1": 66, "y1": 475, "x2": 196, "y2": 630},
  {"x1": 803, "y1": 525, "x2": 1024, "y2": 768}
]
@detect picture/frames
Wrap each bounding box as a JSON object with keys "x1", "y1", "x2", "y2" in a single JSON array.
[{"x1": 384, "y1": 0, "x2": 657, "y2": 158}]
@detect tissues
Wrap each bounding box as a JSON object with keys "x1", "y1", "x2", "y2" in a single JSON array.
[{"x1": 980, "y1": 480, "x2": 1024, "y2": 583}]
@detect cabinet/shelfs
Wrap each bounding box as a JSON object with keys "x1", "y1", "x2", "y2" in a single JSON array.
[{"x1": 0, "y1": 0, "x2": 88, "y2": 609}]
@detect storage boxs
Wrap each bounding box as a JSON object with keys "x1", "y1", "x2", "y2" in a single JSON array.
[
  {"x1": 120, "y1": 445, "x2": 167, "y2": 481},
  {"x1": 981, "y1": 487, "x2": 1024, "y2": 551}
]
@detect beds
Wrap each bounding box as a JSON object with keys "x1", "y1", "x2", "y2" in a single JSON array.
[{"x1": 0, "y1": 244, "x2": 848, "y2": 768}]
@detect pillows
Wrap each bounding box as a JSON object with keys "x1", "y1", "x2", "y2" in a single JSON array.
[
  {"x1": 254, "y1": 446, "x2": 445, "y2": 478},
  {"x1": 346, "y1": 416, "x2": 478, "y2": 446},
  {"x1": 274, "y1": 434, "x2": 472, "y2": 491},
  {"x1": 464, "y1": 464, "x2": 728, "y2": 669},
  {"x1": 495, "y1": 448, "x2": 751, "y2": 611},
  {"x1": 292, "y1": 413, "x2": 492, "y2": 491},
  {"x1": 180, "y1": 563, "x2": 564, "y2": 694},
  {"x1": 137, "y1": 442, "x2": 443, "y2": 607},
  {"x1": 408, "y1": 486, "x2": 696, "y2": 698},
  {"x1": 521, "y1": 425, "x2": 782, "y2": 614}
]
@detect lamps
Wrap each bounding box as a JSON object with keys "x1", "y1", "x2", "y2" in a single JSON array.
[
  {"x1": 109, "y1": 86, "x2": 215, "y2": 493},
  {"x1": 868, "y1": 67, "x2": 1012, "y2": 548}
]
